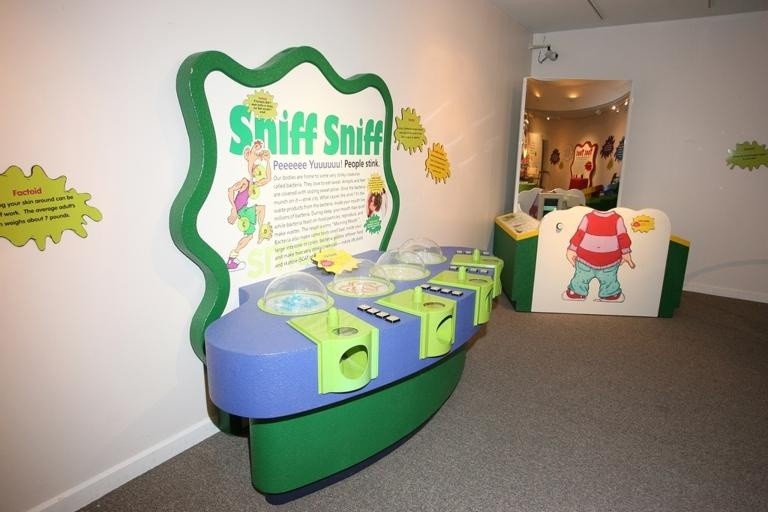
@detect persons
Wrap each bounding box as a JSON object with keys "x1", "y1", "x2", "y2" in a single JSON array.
[
  {"x1": 367, "y1": 193, "x2": 383, "y2": 222},
  {"x1": 560, "y1": 211, "x2": 635, "y2": 304},
  {"x1": 222, "y1": 140, "x2": 274, "y2": 272}
]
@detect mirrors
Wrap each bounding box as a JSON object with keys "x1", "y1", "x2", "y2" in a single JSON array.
[{"x1": 513, "y1": 77, "x2": 633, "y2": 219}]
